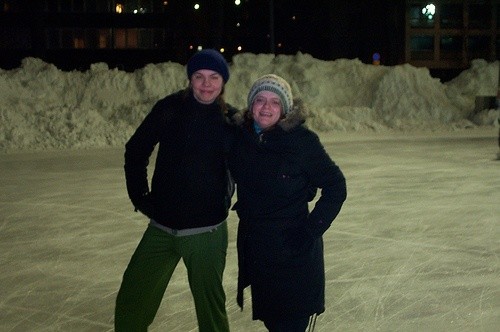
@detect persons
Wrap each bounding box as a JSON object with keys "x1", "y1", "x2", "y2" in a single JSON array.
[
  {"x1": 225, "y1": 72, "x2": 348, "y2": 332},
  {"x1": 113, "y1": 49, "x2": 242, "y2": 332}
]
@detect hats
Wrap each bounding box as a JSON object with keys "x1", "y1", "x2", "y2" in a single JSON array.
[
  {"x1": 247, "y1": 74, "x2": 293, "y2": 114},
  {"x1": 187, "y1": 49, "x2": 230, "y2": 84}
]
